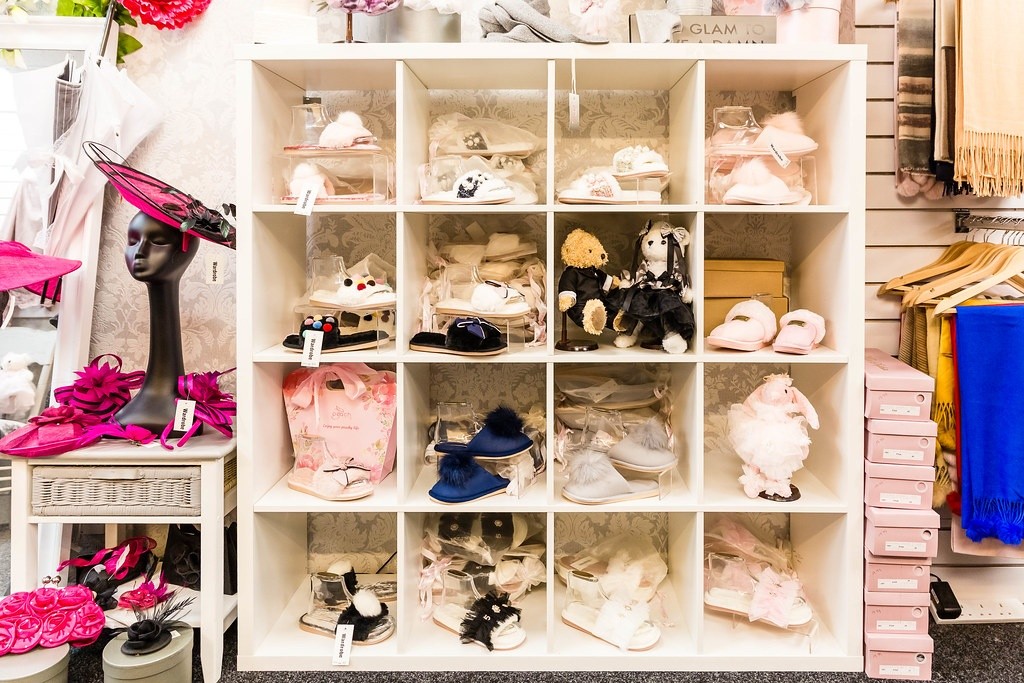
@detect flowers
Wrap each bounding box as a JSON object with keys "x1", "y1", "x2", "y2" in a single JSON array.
[{"x1": 57, "y1": 0, "x2": 212, "y2": 64}]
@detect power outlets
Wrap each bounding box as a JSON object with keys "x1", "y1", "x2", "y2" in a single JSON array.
[{"x1": 937, "y1": 598, "x2": 1024, "y2": 620}]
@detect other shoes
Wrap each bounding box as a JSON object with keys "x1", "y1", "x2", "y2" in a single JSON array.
[
  {"x1": 707, "y1": 300, "x2": 776, "y2": 349},
  {"x1": 772, "y1": 310, "x2": 825, "y2": 354}
]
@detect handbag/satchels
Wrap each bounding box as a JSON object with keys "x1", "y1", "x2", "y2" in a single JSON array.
[{"x1": 282, "y1": 363, "x2": 398, "y2": 484}]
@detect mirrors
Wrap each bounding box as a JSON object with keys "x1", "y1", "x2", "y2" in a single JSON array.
[{"x1": 0, "y1": 15, "x2": 120, "y2": 596}]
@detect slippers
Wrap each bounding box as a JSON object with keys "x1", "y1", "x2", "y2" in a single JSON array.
[
  {"x1": 299, "y1": 590, "x2": 394, "y2": 645},
  {"x1": 702, "y1": 525, "x2": 787, "y2": 571},
  {"x1": 703, "y1": 567, "x2": 812, "y2": 628},
  {"x1": 558, "y1": 557, "x2": 656, "y2": 602},
  {"x1": 287, "y1": 457, "x2": 373, "y2": 500},
  {"x1": 434, "y1": 407, "x2": 534, "y2": 459},
  {"x1": 592, "y1": 421, "x2": 679, "y2": 473},
  {"x1": 435, "y1": 280, "x2": 531, "y2": 318},
  {"x1": 282, "y1": 315, "x2": 389, "y2": 353},
  {"x1": 562, "y1": 452, "x2": 659, "y2": 505},
  {"x1": 281, "y1": 108, "x2": 819, "y2": 205},
  {"x1": 409, "y1": 316, "x2": 508, "y2": 356},
  {"x1": 429, "y1": 454, "x2": 512, "y2": 505},
  {"x1": 432, "y1": 590, "x2": 526, "y2": 650},
  {"x1": 310, "y1": 272, "x2": 396, "y2": 310},
  {"x1": 313, "y1": 561, "x2": 398, "y2": 605},
  {"x1": 561, "y1": 599, "x2": 660, "y2": 650}
]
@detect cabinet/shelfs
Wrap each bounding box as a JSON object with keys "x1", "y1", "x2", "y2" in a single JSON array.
[{"x1": 233, "y1": 46, "x2": 867, "y2": 672}]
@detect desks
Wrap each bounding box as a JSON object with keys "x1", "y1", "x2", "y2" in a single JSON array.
[{"x1": 28, "y1": 415, "x2": 237, "y2": 683}]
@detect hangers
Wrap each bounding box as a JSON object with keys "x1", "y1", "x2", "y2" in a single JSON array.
[{"x1": 877, "y1": 216, "x2": 1024, "y2": 317}]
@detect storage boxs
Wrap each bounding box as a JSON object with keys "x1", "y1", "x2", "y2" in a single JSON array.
[
  {"x1": 629, "y1": 12, "x2": 777, "y2": 44},
  {"x1": 103, "y1": 622, "x2": 194, "y2": 682},
  {"x1": 704, "y1": 259, "x2": 789, "y2": 341},
  {"x1": 864, "y1": 348, "x2": 940, "y2": 683},
  {"x1": 1, "y1": 643, "x2": 70, "y2": 683}
]
